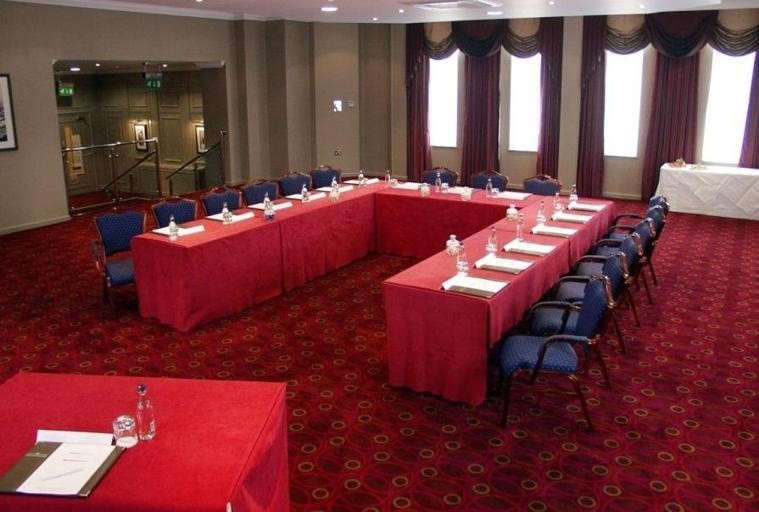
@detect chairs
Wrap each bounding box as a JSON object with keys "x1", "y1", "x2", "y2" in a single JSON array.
[
  {"x1": 312, "y1": 163, "x2": 341, "y2": 187},
  {"x1": 420, "y1": 165, "x2": 458, "y2": 185},
  {"x1": 91, "y1": 210, "x2": 146, "y2": 304},
  {"x1": 244, "y1": 178, "x2": 279, "y2": 204},
  {"x1": 523, "y1": 174, "x2": 564, "y2": 196},
  {"x1": 198, "y1": 184, "x2": 244, "y2": 216},
  {"x1": 470, "y1": 170, "x2": 509, "y2": 190},
  {"x1": 278, "y1": 171, "x2": 313, "y2": 193},
  {"x1": 150, "y1": 194, "x2": 197, "y2": 228}
]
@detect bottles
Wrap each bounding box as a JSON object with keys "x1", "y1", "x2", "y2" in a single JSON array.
[
  {"x1": 357, "y1": 169, "x2": 364, "y2": 186},
  {"x1": 264, "y1": 191, "x2": 270, "y2": 207},
  {"x1": 420, "y1": 183, "x2": 430, "y2": 197},
  {"x1": 455, "y1": 242, "x2": 469, "y2": 278},
  {"x1": 302, "y1": 182, "x2": 308, "y2": 203},
  {"x1": 222, "y1": 201, "x2": 229, "y2": 226},
  {"x1": 552, "y1": 192, "x2": 563, "y2": 213},
  {"x1": 485, "y1": 178, "x2": 493, "y2": 197},
  {"x1": 385, "y1": 170, "x2": 391, "y2": 185},
  {"x1": 505, "y1": 204, "x2": 520, "y2": 221},
  {"x1": 568, "y1": 184, "x2": 580, "y2": 205},
  {"x1": 486, "y1": 226, "x2": 499, "y2": 256},
  {"x1": 436, "y1": 170, "x2": 443, "y2": 192},
  {"x1": 136, "y1": 385, "x2": 158, "y2": 441},
  {"x1": 535, "y1": 200, "x2": 546, "y2": 225},
  {"x1": 446, "y1": 235, "x2": 461, "y2": 256},
  {"x1": 517, "y1": 213, "x2": 527, "y2": 241},
  {"x1": 169, "y1": 214, "x2": 178, "y2": 241}
]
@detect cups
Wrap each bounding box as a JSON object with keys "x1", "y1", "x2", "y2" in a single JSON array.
[
  {"x1": 111, "y1": 416, "x2": 138, "y2": 449},
  {"x1": 441, "y1": 182, "x2": 448, "y2": 193},
  {"x1": 390, "y1": 178, "x2": 398, "y2": 189}
]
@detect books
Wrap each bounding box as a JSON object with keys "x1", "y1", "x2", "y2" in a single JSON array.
[
  {"x1": 0, "y1": 441, "x2": 128, "y2": 498},
  {"x1": 395, "y1": 181, "x2": 533, "y2": 201},
  {"x1": 447, "y1": 201, "x2": 605, "y2": 299},
  {"x1": 151, "y1": 178, "x2": 373, "y2": 237}
]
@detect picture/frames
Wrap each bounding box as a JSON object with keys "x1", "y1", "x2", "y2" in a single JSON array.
[
  {"x1": 134, "y1": 123, "x2": 148, "y2": 152},
  {"x1": 0, "y1": 72, "x2": 18, "y2": 151},
  {"x1": 194, "y1": 124, "x2": 206, "y2": 153}
]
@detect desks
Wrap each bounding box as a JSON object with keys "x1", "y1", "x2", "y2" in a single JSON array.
[
  {"x1": 379, "y1": 170, "x2": 556, "y2": 257},
  {"x1": 132, "y1": 177, "x2": 377, "y2": 334},
  {"x1": 382, "y1": 194, "x2": 616, "y2": 403},
  {"x1": 0, "y1": 371, "x2": 292, "y2": 512},
  {"x1": 652, "y1": 160, "x2": 759, "y2": 220}
]
